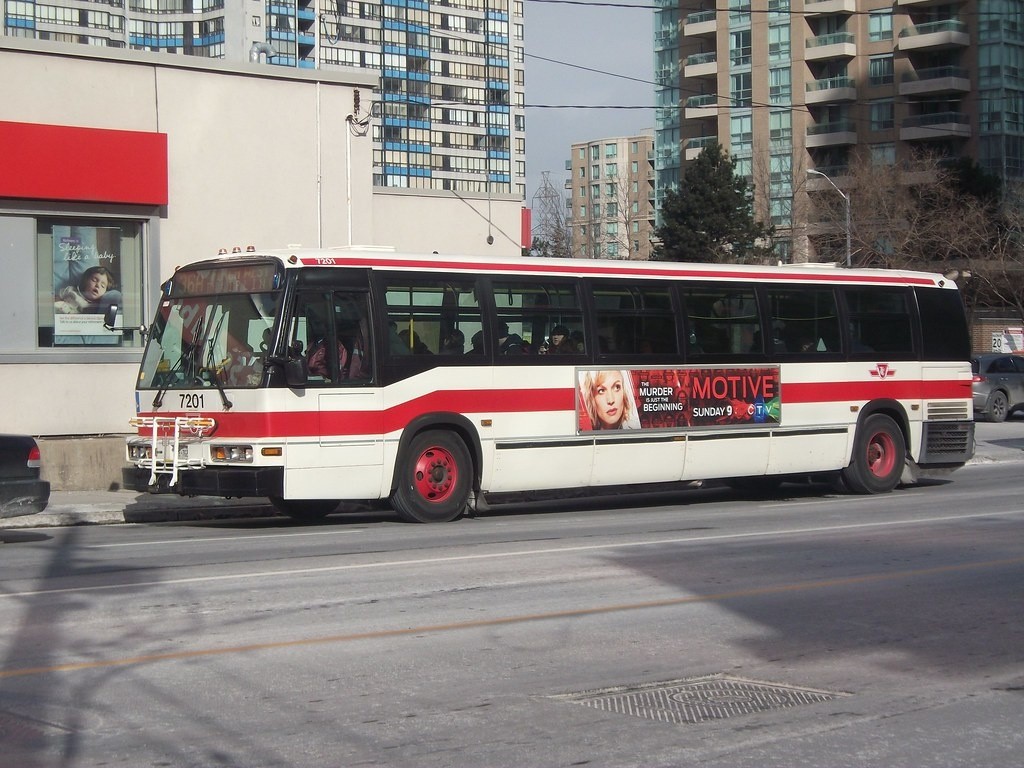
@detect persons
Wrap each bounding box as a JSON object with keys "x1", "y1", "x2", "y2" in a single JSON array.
[
  {"x1": 222, "y1": 298, "x2": 853, "y2": 389},
  {"x1": 579, "y1": 370, "x2": 642, "y2": 430},
  {"x1": 55, "y1": 266, "x2": 115, "y2": 315}
]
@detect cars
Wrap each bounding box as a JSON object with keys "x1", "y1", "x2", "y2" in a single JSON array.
[
  {"x1": 0, "y1": 434, "x2": 50, "y2": 518},
  {"x1": 968, "y1": 352, "x2": 1024, "y2": 422}
]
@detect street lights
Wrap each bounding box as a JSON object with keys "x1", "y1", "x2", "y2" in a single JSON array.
[{"x1": 805, "y1": 168, "x2": 851, "y2": 269}]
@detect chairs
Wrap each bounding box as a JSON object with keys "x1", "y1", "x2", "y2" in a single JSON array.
[{"x1": 572, "y1": 330, "x2": 585, "y2": 354}]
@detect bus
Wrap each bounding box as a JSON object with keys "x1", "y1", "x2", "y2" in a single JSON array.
[{"x1": 104, "y1": 245, "x2": 975, "y2": 524}]
